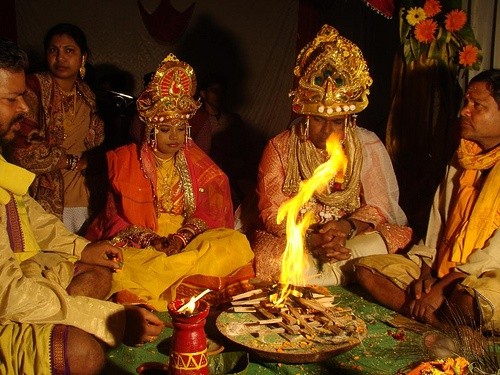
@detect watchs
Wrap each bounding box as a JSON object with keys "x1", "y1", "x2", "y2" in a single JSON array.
[{"x1": 344, "y1": 217, "x2": 357, "y2": 241}]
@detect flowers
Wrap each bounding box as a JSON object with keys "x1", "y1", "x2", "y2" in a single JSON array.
[{"x1": 399, "y1": 0, "x2": 484, "y2": 81}]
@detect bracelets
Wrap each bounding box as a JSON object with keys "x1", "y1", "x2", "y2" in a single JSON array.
[
  {"x1": 66, "y1": 154, "x2": 81, "y2": 170},
  {"x1": 306, "y1": 232, "x2": 313, "y2": 251}
]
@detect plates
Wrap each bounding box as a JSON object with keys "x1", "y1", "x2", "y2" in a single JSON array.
[{"x1": 216, "y1": 306, "x2": 367, "y2": 363}]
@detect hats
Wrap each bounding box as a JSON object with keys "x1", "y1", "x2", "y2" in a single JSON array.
[
  {"x1": 136, "y1": 53, "x2": 204, "y2": 147},
  {"x1": 290, "y1": 24, "x2": 373, "y2": 116}
]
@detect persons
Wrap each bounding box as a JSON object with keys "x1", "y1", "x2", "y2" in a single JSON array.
[
  {"x1": 349, "y1": 69, "x2": 500, "y2": 337},
  {"x1": 96, "y1": 54, "x2": 250, "y2": 375},
  {"x1": 253, "y1": 23, "x2": 418, "y2": 285},
  {"x1": 4, "y1": 22, "x2": 104, "y2": 237},
  {"x1": 0, "y1": 45, "x2": 165, "y2": 375}
]
